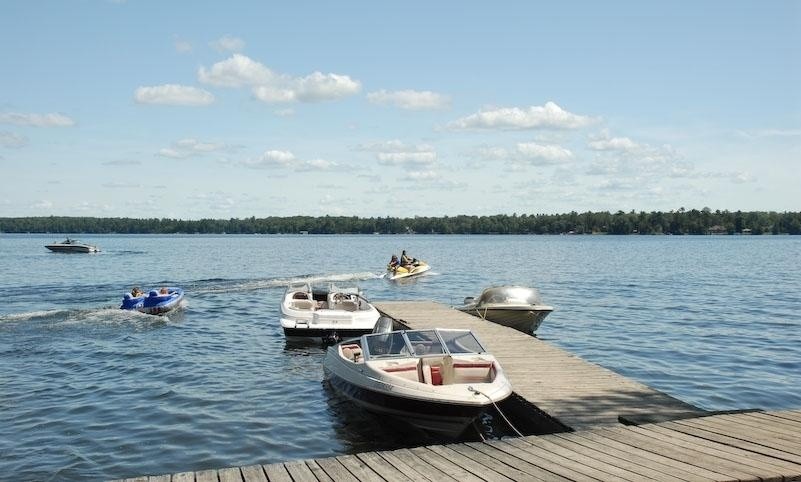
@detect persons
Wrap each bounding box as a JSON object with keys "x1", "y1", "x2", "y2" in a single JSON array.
[
  {"x1": 401, "y1": 250, "x2": 416, "y2": 273},
  {"x1": 387, "y1": 255, "x2": 401, "y2": 273},
  {"x1": 160, "y1": 288, "x2": 169, "y2": 296},
  {"x1": 132, "y1": 287, "x2": 144, "y2": 298}
]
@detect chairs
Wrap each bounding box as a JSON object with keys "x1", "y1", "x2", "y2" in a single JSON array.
[
  {"x1": 290, "y1": 292, "x2": 359, "y2": 311},
  {"x1": 374, "y1": 354, "x2": 494, "y2": 384},
  {"x1": 122, "y1": 290, "x2": 172, "y2": 308}
]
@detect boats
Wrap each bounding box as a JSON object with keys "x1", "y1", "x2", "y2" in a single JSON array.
[
  {"x1": 278, "y1": 280, "x2": 379, "y2": 346},
  {"x1": 376, "y1": 257, "x2": 429, "y2": 282},
  {"x1": 43, "y1": 239, "x2": 100, "y2": 253},
  {"x1": 120, "y1": 285, "x2": 185, "y2": 315},
  {"x1": 321, "y1": 316, "x2": 514, "y2": 439},
  {"x1": 452, "y1": 282, "x2": 554, "y2": 335}
]
